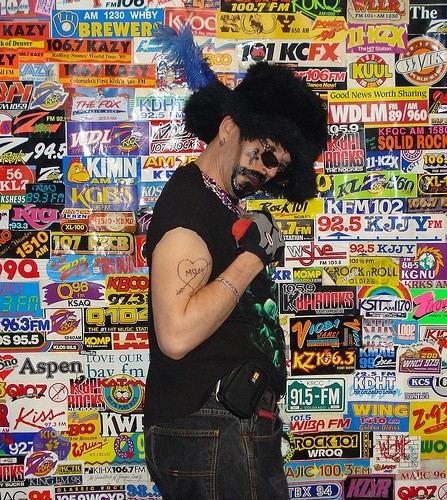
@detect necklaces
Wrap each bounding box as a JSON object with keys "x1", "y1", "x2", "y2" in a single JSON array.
[{"x1": 202, "y1": 171, "x2": 240, "y2": 215}]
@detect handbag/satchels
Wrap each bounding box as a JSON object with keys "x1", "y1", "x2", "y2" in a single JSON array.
[{"x1": 217, "y1": 360, "x2": 270, "y2": 419}]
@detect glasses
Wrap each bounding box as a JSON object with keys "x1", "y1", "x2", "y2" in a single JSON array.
[{"x1": 258, "y1": 138, "x2": 290, "y2": 183}]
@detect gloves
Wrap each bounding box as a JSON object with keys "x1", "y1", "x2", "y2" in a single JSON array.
[{"x1": 232, "y1": 205, "x2": 285, "y2": 267}]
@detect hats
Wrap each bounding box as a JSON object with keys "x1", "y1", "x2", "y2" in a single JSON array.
[{"x1": 151, "y1": 21, "x2": 327, "y2": 204}]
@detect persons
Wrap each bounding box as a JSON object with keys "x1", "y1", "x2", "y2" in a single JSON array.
[{"x1": 140, "y1": 62, "x2": 330, "y2": 500}]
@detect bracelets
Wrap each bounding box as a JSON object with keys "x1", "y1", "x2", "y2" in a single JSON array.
[{"x1": 215, "y1": 277, "x2": 239, "y2": 303}]
